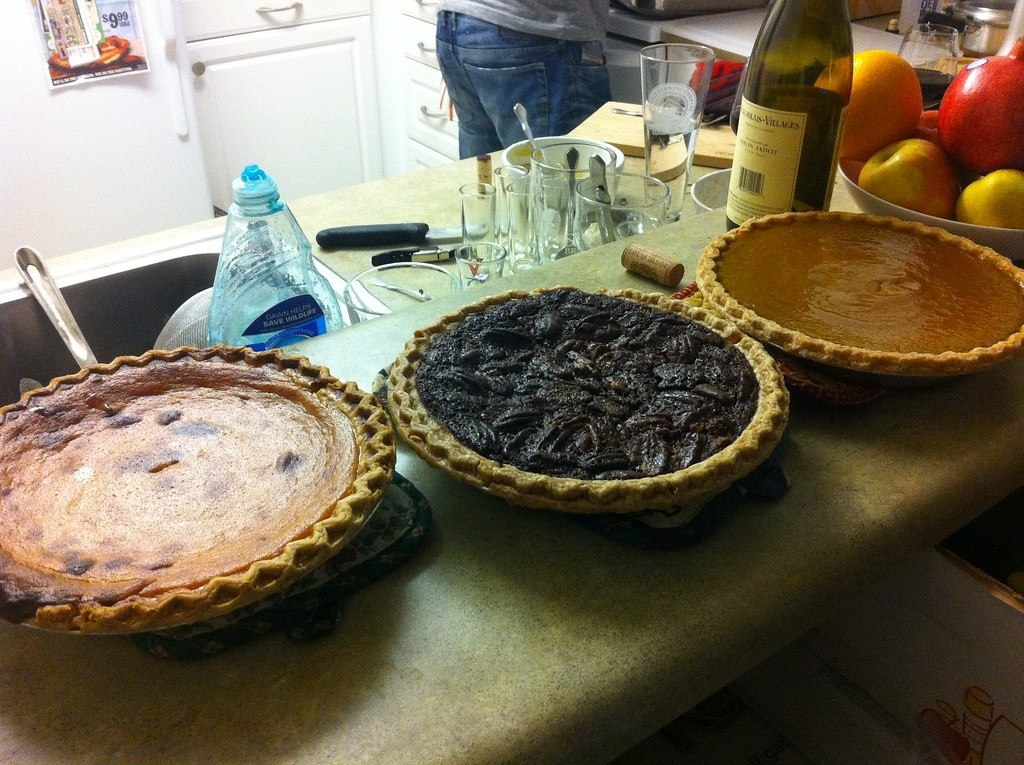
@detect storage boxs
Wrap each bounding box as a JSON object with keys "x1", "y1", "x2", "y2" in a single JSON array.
[{"x1": 730, "y1": 544, "x2": 1024, "y2": 765}]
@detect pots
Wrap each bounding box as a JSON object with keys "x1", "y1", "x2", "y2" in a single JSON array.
[{"x1": 915, "y1": 0, "x2": 1018, "y2": 57}]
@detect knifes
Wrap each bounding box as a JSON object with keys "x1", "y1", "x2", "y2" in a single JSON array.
[{"x1": 316, "y1": 223, "x2": 490, "y2": 246}]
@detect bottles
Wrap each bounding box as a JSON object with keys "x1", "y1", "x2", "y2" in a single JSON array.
[
  {"x1": 208, "y1": 164, "x2": 341, "y2": 353},
  {"x1": 725, "y1": 0, "x2": 853, "y2": 231}
]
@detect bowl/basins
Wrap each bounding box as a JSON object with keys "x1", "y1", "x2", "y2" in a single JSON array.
[
  {"x1": 691, "y1": 168, "x2": 732, "y2": 213},
  {"x1": 839, "y1": 164, "x2": 1024, "y2": 262},
  {"x1": 501, "y1": 136, "x2": 626, "y2": 198}
]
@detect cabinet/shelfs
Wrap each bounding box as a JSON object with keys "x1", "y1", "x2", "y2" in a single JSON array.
[
  {"x1": 176, "y1": 0, "x2": 385, "y2": 214},
  {"x1": 397, "y1": 0, "x2": 460, "y2": 172}
]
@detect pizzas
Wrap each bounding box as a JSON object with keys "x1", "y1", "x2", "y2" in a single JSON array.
[
  {"x1": 385, "y1": 283, "x2": 792, "y2": 513},
  {"x1": 695, "y1": 211, "x2": 1024, "y2": 374},
  {"x1": 0, "y1": 346, "x2": 398, "y2": 637}
]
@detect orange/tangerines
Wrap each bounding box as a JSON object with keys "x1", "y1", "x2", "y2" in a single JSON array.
[{"x1": 815, "y1": 50, "x2": 922, "y2": 163}]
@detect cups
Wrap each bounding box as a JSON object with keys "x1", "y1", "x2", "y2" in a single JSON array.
[
  {"x1": 530, "y1": 143, "x2": 617, "y2": 263},
  {"x1": 508, "y1": 183, "x2": 544, "y2": 273},
  {"x1": 344, "y1": 262, "x2": 463, "y2": 325},
  {"x1": 576, "y1": 173, "x2": 669, "y2": 253},
  {"x1": 898, "y1": 24, "x2": 958, "y2": 108},
  {"x1": 494, "y1": 165, "x2": 528, "y2": 233},
  {"x1": 461, "y1": 182, "x2": 496, "y2": 243},
  {"x1": 456, "y1": 244, "x2": 507, "y2": 289},
  {"x1": 640, "y1": 43, "x2": 715, "y2": 222}
]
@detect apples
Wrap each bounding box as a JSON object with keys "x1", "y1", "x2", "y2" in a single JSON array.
[{"x1": 845, "y1": 106, "x2": 1024, "y2": 230}]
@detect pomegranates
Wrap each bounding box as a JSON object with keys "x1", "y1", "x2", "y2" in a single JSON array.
[{"x1": 933, "y1": 38, "x2": 1024, "y2": 173}]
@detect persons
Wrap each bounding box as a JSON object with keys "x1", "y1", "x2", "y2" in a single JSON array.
[{"x1": 436, "y1": 0, "x2": 612, "y2": 160}]
